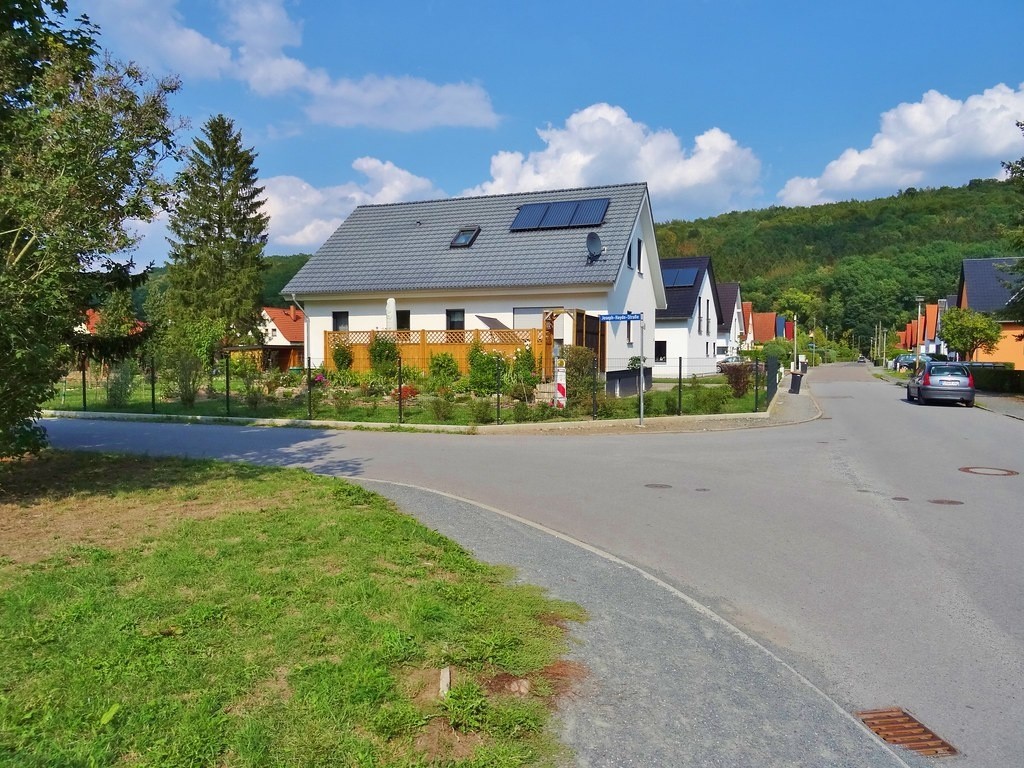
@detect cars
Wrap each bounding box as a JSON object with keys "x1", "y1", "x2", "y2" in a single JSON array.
[
  {"x1": 906, "y1": 361, "x2": 976, "y2": 408},
  {"x1": 893, "y1": 353, "x2": 940, "y2": 373},
  {"x1": 858, "y1": 356, "x2": 865, "y2": 364},
  {"x1": 716, "y1": 356, "x2": 752, "y2": 373}
]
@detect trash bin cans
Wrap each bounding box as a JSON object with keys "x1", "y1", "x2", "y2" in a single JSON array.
[
  {"x1": 800, "y1": 361, "x2": 808, "y2": 373},
  {"x1": 788, "y1": 372, "x2": 804, "y2": 394},
  {"x1": 289, "y1": 366, "x2": 305, "y2": 377}
]
